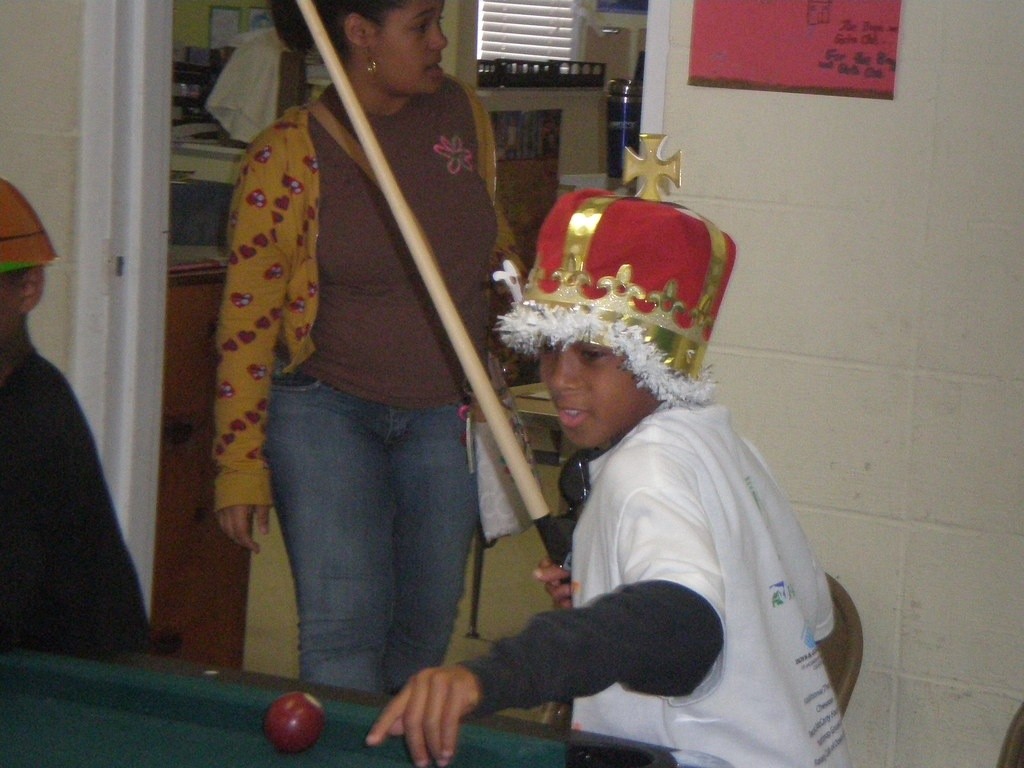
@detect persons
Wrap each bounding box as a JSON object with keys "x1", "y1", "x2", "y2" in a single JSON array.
[
  {"x1": 1, "y1": 179, "x2": 157, "y2": 663},
  {"x1": 365, "y1": 131, "x2": 851, "y2": 768},
  {"x1": 210, "y1": 0, "x2": 534, "y2": 704}
]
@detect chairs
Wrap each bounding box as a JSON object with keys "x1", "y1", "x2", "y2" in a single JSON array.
[{"x1": 538, "y1": 573, "x2": 864, "y2": 725}]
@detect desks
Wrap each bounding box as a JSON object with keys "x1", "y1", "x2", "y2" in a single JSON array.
[
  {"x1": 0, "y1": 633, "x2": 670, "y2": 768},
  {"x1": 461, "y1": 388, "x2": 615, "y2": 642}
]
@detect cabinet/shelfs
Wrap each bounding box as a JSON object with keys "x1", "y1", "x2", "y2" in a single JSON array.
[{"x1": 151, "y1": 248, "x2": 255, "y2": 668}]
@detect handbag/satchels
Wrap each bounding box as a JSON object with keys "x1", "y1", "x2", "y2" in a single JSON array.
[{"x1": 467, "y1": 393, "x2": 571, "y2": 543}]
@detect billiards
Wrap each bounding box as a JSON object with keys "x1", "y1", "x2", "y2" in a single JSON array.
[{"x1": 262, "y1": 690, "x2": 326, "y2": 754}]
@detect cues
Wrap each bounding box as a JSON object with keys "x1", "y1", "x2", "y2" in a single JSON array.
[{"x1": 293, "y1": 0, "x2": 567, "y2": 564}]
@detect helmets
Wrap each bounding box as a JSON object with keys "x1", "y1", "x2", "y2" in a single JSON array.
[{"x1": 0, "y1": 176, "x2": 58, "y2": 269}]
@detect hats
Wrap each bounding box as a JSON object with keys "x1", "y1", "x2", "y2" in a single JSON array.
[{"x1": 494, "y1": 130, "x2": 737, "y2": 404}]
